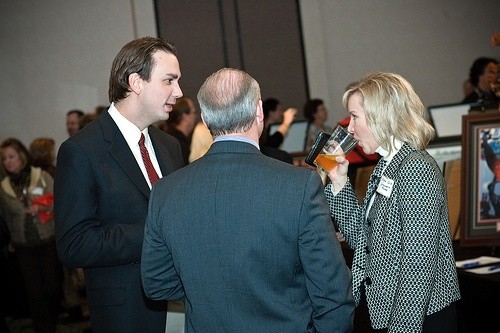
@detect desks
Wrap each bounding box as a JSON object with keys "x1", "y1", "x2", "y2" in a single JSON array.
[{"x1": 451, "y1": 241, "x2": 500, "y2": 333}]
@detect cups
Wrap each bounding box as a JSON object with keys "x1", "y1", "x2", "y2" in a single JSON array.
[{"x1": 313, "y1": 125, "x2": 358, "y2": 175}]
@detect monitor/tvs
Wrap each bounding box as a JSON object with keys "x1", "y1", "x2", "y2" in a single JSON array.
[
  {"x1": 269, "y1": 120, "x2": 310, "y2": 155},
  {"x1": 428, "y1": 102, "x2": 471, "y2": 143}
]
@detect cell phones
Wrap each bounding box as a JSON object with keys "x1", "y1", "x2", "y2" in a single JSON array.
[{"x1": 305, "y1": 131, "x2": 331, "y2": 168}]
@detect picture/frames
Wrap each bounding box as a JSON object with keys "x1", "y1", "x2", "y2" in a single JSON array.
[{"x1": 460, "y1": 111, "x2": 500, "y2": 247}]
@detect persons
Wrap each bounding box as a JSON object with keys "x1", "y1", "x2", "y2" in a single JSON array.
[
  {"x1": 479, "y1": 139, "x2": 500, "y2": 219},
  {"x1": 141, "y1": 68, "x2": 356, "y2": 333},
  {"x1": 0, "y1": 104, "x2": 112, "y2": 333},
  {"x1": 151, "y1": 96, "x2": 214, "y2": 164},
  {"x1": 54, "y1": 36, "x2": 190, "y2": 333},
  {"x1": 258, "y1": 98, "x2": 379, "y2": 243},
  {"x1": 324, "y1": 72, "x2": 461, "y2": 333},
  {"x1": 462, "y1": 57, "x2": 500, "y2": 103}
]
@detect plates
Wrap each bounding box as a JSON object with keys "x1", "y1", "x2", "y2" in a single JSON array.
[{"x1": 456, "y1": 256, "x2": 500, "y2": 274}]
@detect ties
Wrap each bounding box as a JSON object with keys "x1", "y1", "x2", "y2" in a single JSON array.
[{"x1": 138, "y1": 134, "x2": 160, "y2": 185}]
